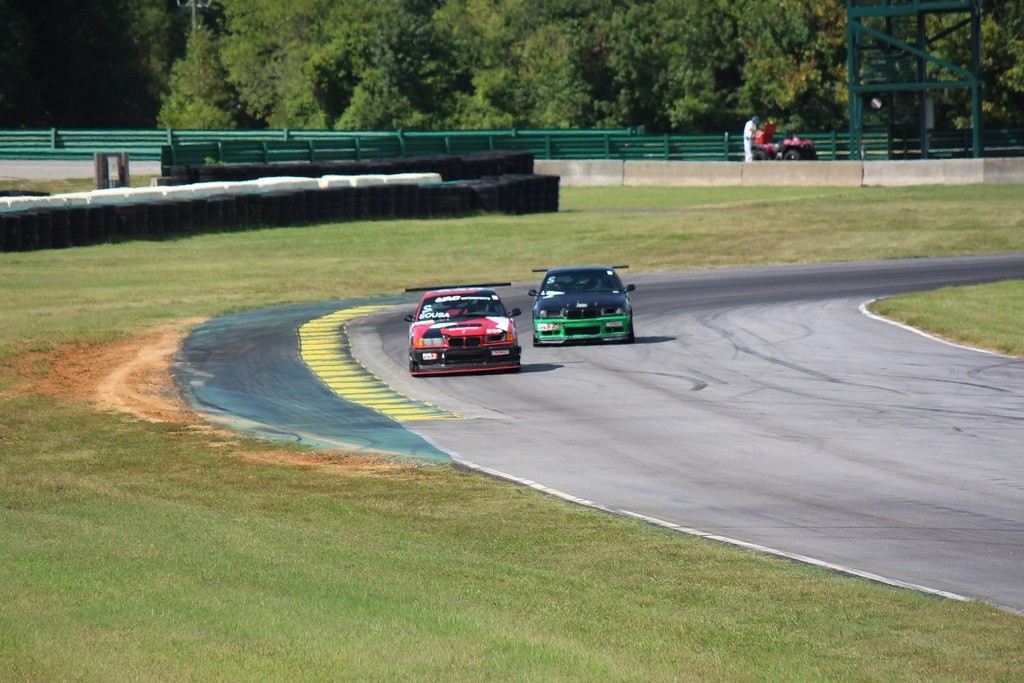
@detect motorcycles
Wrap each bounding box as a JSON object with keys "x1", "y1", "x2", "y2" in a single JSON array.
[{"x1": 750, "y1": 120, "x2": 818, "y2": 161}]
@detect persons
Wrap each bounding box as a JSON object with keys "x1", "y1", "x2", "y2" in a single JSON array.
[
  {"x1": 594, "y1": 278, "x2": 608, "y2": 288},
  {"x1": 744, "y1": 115, "x2": 759, "y2": 161},
  {"x1": 551, "y1": 281, "x2": 566, "y2": 290},
  {"x1": 472, "y1": 300, "x2": 490, "y2": 312}
]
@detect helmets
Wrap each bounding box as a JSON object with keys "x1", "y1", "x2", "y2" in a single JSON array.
[
  {"x1": 473, "y1": 299, "x2": 487, "y2": 312},
  {"x1": 752, "y1": 116, "x2": 759, "y2": 123}
]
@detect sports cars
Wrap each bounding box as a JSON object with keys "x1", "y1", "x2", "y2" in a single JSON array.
[
  {"x1": 401, "y1": 282, "x2": 523, "y2": 377},
  {"x1": 527, "y1": 262, "x2": 637, "y2": 347}
]
